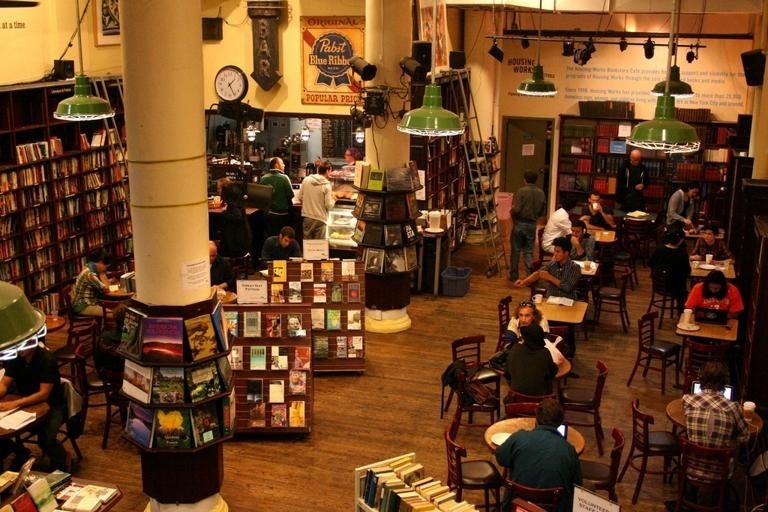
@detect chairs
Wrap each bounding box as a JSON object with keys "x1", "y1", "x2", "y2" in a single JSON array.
[
  {"x1": 665, "y1": 192, "x2": 768, "y2": 512},
  {"x1": 505, "y1": 479, "x2": 563, "y2": 512},
  {"x1": 625, "y1": 309, "x2": 682, "y2": 394},
  {"x1": 445, "y1": 213, "x2": 640, "y2": 455},
  {"x1": 446, "y1": 431, "x2": 505, "y2": 512},
  {"x1": 644, "y1": 273, "x2": 682, "y2": 331},
  {"x1": 615, "y1": 400, "x2": 681, "y2": 504},
  {"x1": 578, "y1": 429, "x2": 623, "y2": 505}
]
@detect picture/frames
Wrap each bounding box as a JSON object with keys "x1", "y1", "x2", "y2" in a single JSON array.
[{"x1": 92, "y1": 0, "x2": 120, "y2": 47}]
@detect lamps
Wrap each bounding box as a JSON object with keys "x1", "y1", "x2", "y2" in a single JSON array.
[
  {"x1": 560, "y1": 27, "x2": 603, "y2": 63},
  {"x1": 52, "y1": 1, "x2": 115, "y2": 121},
  {"x1": 625, "y1": 2, "x2": 701, "y2": 153},
  {"x1": 349, "y1": 55, "x2": 425, "y2": 131},
  {"x1": 654, "y1": 3, "x2": 692, "y2": 99},
  {"x1": 206, "y1": 101, "x2": 273, "y2": 209},
  {"x1": 619, "y1": 36, "x2": 628, "y2": 53},
  {"x1": 644, "y1": 37, "x2": 656, "y2": 59},
  {"x1": 488, "y1": 41, "x2": 508, "y2": 64},
  {"x1": 396, "y1": 0, "x2": 465, "y2": 138},
  {"x1": 518, "y1": 2, "x2": 558, "y2": 99},
  {"x1": 686, "y1": 51, "x2": 698, "y2": 64}
]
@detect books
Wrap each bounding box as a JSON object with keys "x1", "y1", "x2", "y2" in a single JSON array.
[
  {"x1": 270, "y1": 259, "x2": 366, "y2": 362},
  {"x1": 545, "y1": 295, "x2": 574, "y2": 307},
  {"x1": 0, "y1": 407, "x2": 36, "y2": 430},
  {"x1": 427, "y1": 111, "x2": 499, "y2": 235},
  {"x1": 351, "y1": 161, "x2": 421, "y2": 274},
  {"x1": 558, "y1": 119, "x2": 734, "y2": 218},
  {"x1": 0, "y1": 458, "x2": 123, "y2": 512},
  {"x1": 117, "y1": 293, "x2": 238, "y2": 449},
  {"x1": 226, "y1": 303, "x2": 307, "y2": 428},
  {"x1": 0, "y1": 123, "x2": 135, "y2": 316}
]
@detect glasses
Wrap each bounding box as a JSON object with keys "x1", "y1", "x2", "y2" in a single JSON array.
[{"x1": 519, "y1": 302, "x2": 533, "y2": 307}]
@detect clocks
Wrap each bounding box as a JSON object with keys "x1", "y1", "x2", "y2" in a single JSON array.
[{"x1": 214, "y1": 65, "x2": 249, "y2": 102}]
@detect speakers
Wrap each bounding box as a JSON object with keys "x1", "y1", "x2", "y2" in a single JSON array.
[
  {"x1": 54, "y1": 59, "x2": 74, "y2": 80},
  {"x1": 203, "y1": 18, "x2": 222, "y2": 40},
  {"x1": 449, "y1": 51, "x2": 465, "y2": 69},
  {"x1": 734, "y1": 115, "x2": 752, "y2": 151},
  {"x1": 413, "y1": 41, "x2": 432, "y2": 72},
  {"x1": 741, "y1": 48, "x2": 766, "y2": 86}
]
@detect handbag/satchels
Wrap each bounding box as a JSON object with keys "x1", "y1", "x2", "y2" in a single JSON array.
[{"x1": 464, "y1": 368, "x2": 492, "y2": 405}]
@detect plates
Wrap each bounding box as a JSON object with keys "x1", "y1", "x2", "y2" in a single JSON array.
[
  {"x1": 425, "y1": 228, "x2": 444, "y2": 233},
  {"x1": 491, "y1": 433, "x2": 512, "y2": 446},
  {"x1": 676, "y1": 322, "x2": 700, "y2": 331},
  {"x1": 699, "y1": 264, "x2": 716, "y2": 270}
]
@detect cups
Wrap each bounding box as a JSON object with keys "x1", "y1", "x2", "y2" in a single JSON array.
[
  {"x1": 584, "y1": 260, "x2": 592, "y2": 271},
  {"x1": 532, "y1": 294, "x2": 543, "y2": 303},
  {"x1": 594, "y1": 229, "x2": 616, "y2": 241},
  {"x1": 705, "y1": 254, "x2": 714, "y2": 264},
  {"x1": 683, "y1": 309, "x2": 693, "y2": 323},
  {"x1": 743, "y1": 401, "x2": 756, "y2": 421},
  {"x1": 592, "y1": 203, "x2": 598, "y2": 210}
]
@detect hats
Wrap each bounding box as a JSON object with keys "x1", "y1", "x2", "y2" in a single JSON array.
[{"x1": 520, "y1": 324, "x2": 545, "y2": 350}]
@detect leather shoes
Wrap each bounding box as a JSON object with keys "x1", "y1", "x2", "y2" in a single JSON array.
[{"x1": 567, "y1": 372, "x2": 579, "y2": 378}]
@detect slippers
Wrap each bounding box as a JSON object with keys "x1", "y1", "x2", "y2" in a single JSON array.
[{"x1": 10, "y1": 447, "x2": 30, "y2": 472}]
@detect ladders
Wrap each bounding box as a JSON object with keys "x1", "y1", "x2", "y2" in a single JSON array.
[
  {"x1": 90, "y1": 74, "x2": 130, "y2": 205},
  {"x1": 449, "y1": 68, "x2": 509, "y2": 271}
]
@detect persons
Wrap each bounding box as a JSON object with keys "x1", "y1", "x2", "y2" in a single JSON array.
[
  {"x1": 361, "y1": 457, "x2": 479, "y2": 512},
  {"x1": 508, "y1": 170, "x2": 546, "y2": 282},
  {"x1": 563, "y1": 221, "x2": 596, "y2": 298},
  {"x1": 689, "y1": 223, "x2": 736, "y2": 264},
  {"x1": 515, "y1": 238, "x2": 580, "y2": 301},
  {"x1": 647, "y1": 229, "x2": 692, "y2": 320},
  {"x1": 664, "y1": 179, "x2": 701, "y2": 239},
  {"x1": 503, "y1": 324, "x2": 559, "y2": 406},
  {"x1": 496, "y1": 397, "x2": 596, "y2": 512},
  {"x1": 580, "y1": 191, "x2": 616, "y2": 286},
  {"x1": 682, "y1": 362, "x2": 751, "y2": 511},
  {"x1": 506, "y1": 300, "x2": 550, "y2": 338},
  {"x1": 209, "y1": 147, "x2": 362, "y2": 293},
  {"x1": 0, "y1": 340, "x2": 69, "y2": 471},
  {"x1": 93, "y1": 303, "x2": 128, "y2": 397},
  {"x1": 542, "y1": 196, "x2": 577, "y2": 253},
  {"x1": 685, "y1": 270, "x2": 745, "y2": 387}
]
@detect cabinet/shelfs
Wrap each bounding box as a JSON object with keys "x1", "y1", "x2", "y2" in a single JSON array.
[
  {"x1": 0, "y1": 187, "x2": 425, "y2": 512},
  {"x1": 555, "y1": 114, "x2": 738, "y2": 224},
  {"x1": 0, "y1": 75, "x2": 136, "y2": 314}
]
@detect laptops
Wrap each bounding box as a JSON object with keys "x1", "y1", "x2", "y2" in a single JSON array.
[
  {"x1": 691, "y1": 381, "x2": 734, "y2": 401},
  {"x1": 536, "y1": 423, "x2": 568, "y2": 438},
  {"x1": 694, "y1": 307, "x2": 728, "y2": 325}
]
